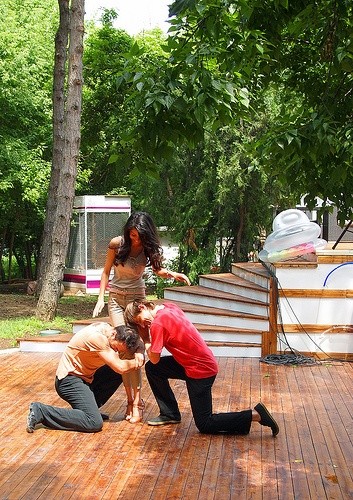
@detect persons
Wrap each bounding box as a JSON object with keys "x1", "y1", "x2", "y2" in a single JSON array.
[
  {"x1": 124, "y1": 299, "x2": 280, "y2": 437},
  {"x1": 92, "y1": 211, "x2": 192, "y2": 423},
  {"x1": 26, "y1": 321, "x2": 145, "y2": 434}
]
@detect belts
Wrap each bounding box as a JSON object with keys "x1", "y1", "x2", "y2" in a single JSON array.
[{"x1": 109, "y1": 288, "x2": 144, "y2": 295}]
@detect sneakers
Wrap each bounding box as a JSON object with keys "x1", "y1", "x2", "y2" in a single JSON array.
[
  {"x1": 148, "y1": 415, "x2": 181, "y2": 425},
  {"x1": 26, "y1": 403, "x2": 36, "y2": 433},
  {"x1": 101, "y1": 414, "x2": 109, "y2": 421},
  {"x1": 254, "y1": 402, "x2": 279, "y2": 436}
]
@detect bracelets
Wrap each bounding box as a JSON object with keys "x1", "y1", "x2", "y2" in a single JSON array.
[{"x1": 167, "y1": 268, "x2": 170, "y2": 280}]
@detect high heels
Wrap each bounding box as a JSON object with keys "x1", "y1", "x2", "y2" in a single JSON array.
[{"x1": 125, "y1": 399, "x2": 145, "y2": 424}]
